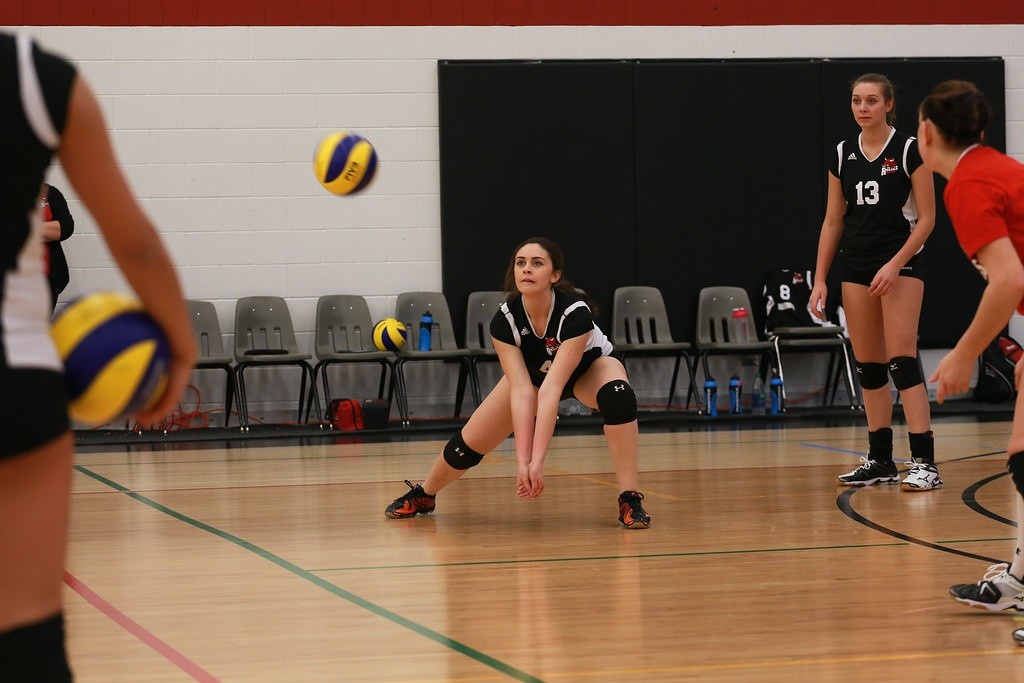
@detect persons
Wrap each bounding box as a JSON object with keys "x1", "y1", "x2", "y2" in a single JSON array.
[
  {"x1": 916, "y1": 79, "x2": 1024, "y2": 648},
  {"x1": 806, "y1": 73, "x2": 946, "y2": 491},
  {"x1": 0, "y1": 27, "x2": 205, "y2": 683},
  {"x1": 39, "y1": 182, "x2": 74, "y2": 316},
  {"x1": 385, "y1": 237, "x2": 652, "y2": 530}
]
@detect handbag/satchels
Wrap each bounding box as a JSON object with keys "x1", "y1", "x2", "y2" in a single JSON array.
[{"x1": 324, "y1": 395, "x2": 388, "y2": 431}]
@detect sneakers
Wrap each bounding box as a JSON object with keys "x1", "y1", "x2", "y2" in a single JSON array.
[
  {"x1": 1012, "y1": 627, "x2": 1024, "y2": 645},
  {"x1": 385, "y1": 480, "x2": 436, "y2": 519},
  {"x1": 948, "y1": 563, "x2": 1024, "y2": 612},
  {"x1": 836, "y1": 451, "x2": 900, "y2": 485},
  {"x1": 617, "y1": 490, "x2": 651, "y2": 529},
  {"x1": 899, "y1": 457, "x2": 943, "y2": 491}
]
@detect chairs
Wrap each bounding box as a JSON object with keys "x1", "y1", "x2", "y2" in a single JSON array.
[
  {"x1": 457, "y1": 292, "x2": 556, "y2": 417},
  {"x1": 229, "y1": 297, "x2": 324, "y2": 429},
  {"x1": 591, "y1": 285, "x2": 703, "y2": 415},
  {"x1": 389, "y1": 293, "x2": 478, "y2": 425},
  {"x1": 770, "y1": 326, "x2": 930, "y2": 412},
  {"x1": 123, "y1": 298, "x2": 244, "y2": 433},
  {"x1": 306, "y1": 295, "x2": 406, "y2": 428},
  {"x1": 686, "y1": 287, "x2": 780, "y2": 414}
]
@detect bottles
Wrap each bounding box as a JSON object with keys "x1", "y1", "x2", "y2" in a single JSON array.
[
  {"x1": 728, "y1": 374, "x2": 742, "y2": 415},
  {"x1": 418, "y1": 311, "x2": 433, "y2": 351},
  {"x1": 752, "y1": 372, "x2": 766, "y2": 415},
  {"x1": 703, "y1": 375, "x2": 717, "y2": 417},
  {"x1": 770, "y1": 373, "x2": 786, "y2": 416}
]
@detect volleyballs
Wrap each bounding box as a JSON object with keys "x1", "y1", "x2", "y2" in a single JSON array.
[
  {"x1": 372, "y1": 317, "x2": 407, "y2": 354},
  {"x1": 310, "y1": 129, "x2": 380, "y2": 197},
  {"x1": 47, "y1": 288, "x2": 179, "y2": 425}
]
